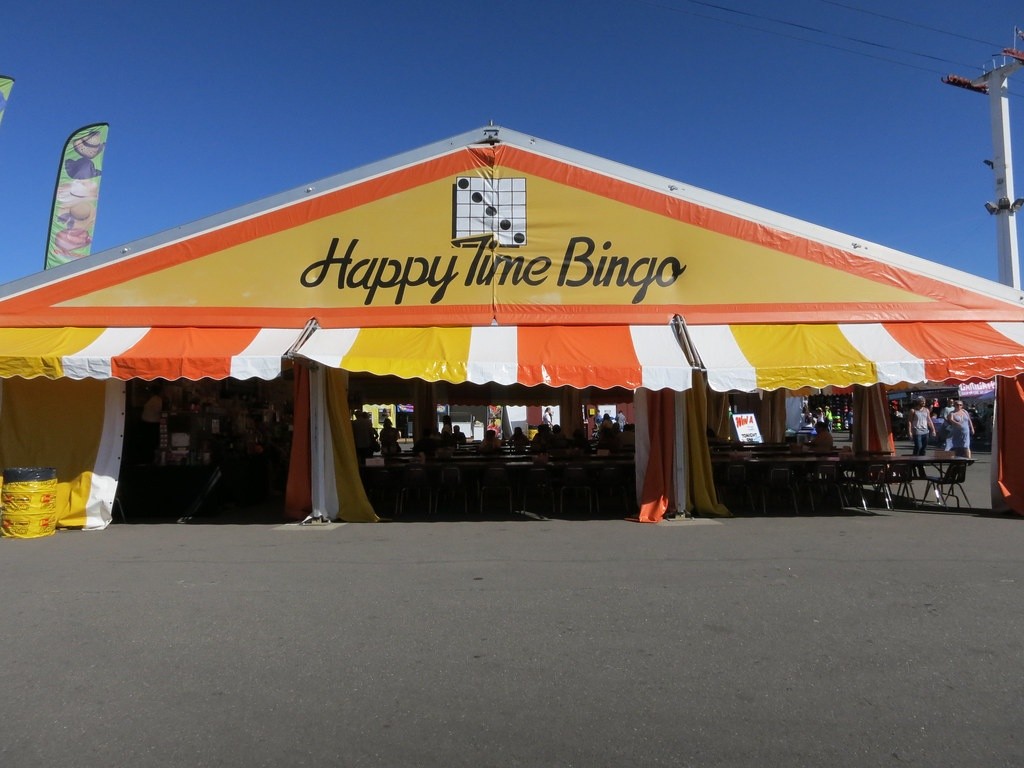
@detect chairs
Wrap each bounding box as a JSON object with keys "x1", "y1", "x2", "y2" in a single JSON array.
[
  {"x1": 720, "y1": 460, "x2": 972, "y2": 512},
  {"x1": 396, "y1": 464, "x2": 626, "y2": 517}
]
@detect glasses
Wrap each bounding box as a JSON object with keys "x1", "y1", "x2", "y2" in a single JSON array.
[{"x1": 956, "y1": 404, "x2": 963, "y2": 406}]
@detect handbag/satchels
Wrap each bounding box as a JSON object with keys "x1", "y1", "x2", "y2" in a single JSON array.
[
  {"x1": 373, "y1": 436, "x2": 380, "y2": 452},
  {"x1": 389, "y1": 441, "x2": 401, "y2": 453}
]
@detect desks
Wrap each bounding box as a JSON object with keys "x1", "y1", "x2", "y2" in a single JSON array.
[
  {"x1": 361, "y1": 441, "x2": 644, "y2": 484},
  {"x1": 707, "y1": 435, "x2": 978, "y2": 490}
]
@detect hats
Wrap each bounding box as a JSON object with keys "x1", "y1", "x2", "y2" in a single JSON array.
[
  {"x1": 818, "y1": 407, "x2": 822, "y2": 411},
  {"x1": 825, "y1": 406, "x2": 830, "y2": 409}
]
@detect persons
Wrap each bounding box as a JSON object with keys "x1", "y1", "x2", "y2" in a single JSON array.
[
  {"x1": 710, "y1": 393, "x2": 993, "y2": 455},
  {"x1": 140, "y1": 384, "x2": 292, "y2": 464},
  {"x1": 351, "y1": 410, "x2": 635, "y2": 494}
]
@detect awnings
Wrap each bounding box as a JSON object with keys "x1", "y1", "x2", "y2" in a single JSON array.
[{"x1": 0, "y1": 321, "x2": 1024, "y2": 392}]
